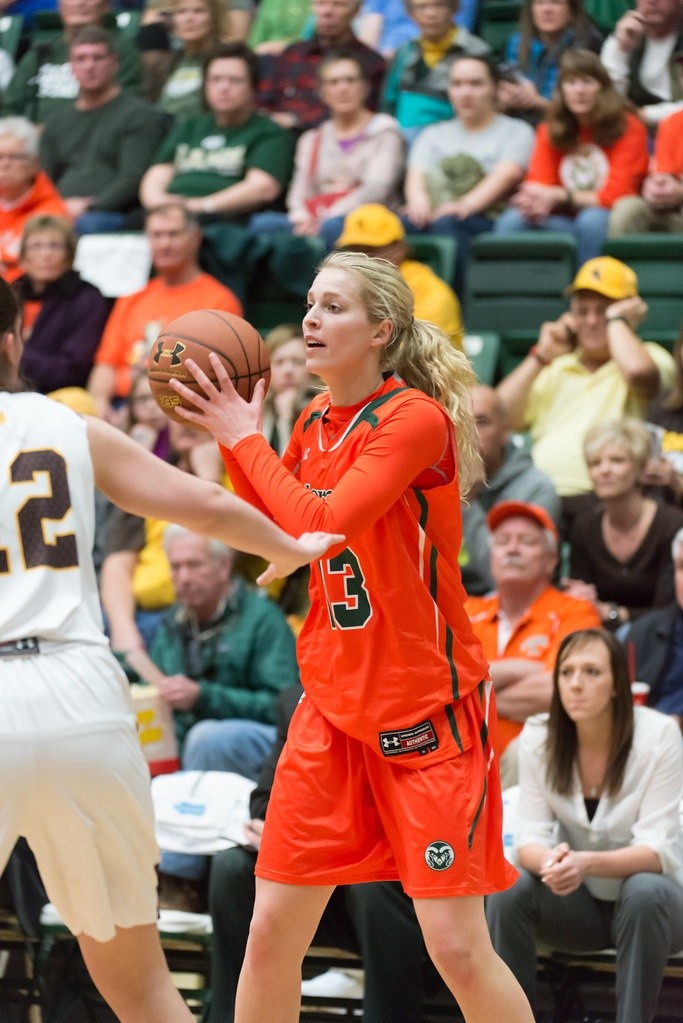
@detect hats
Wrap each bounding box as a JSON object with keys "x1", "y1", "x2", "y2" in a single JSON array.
[
  {"x1": 334, "y1": 203, "x2": 405, "y2": 251},
  {"x1": 488, "y1": 503, "x2": 559, "y2": 545},
  {"x1": 565, "y1": 255, "x2": 638, "y2": 300}
]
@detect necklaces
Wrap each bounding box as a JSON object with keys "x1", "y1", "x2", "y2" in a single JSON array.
[{"x1": 582, "y1": 780, "x2": 603, "y2": 797}]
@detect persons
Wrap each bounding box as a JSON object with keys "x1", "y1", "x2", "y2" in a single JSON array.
[
  {"x1": 0, "y1": 0, "x2": 683, "y2": 1023},
  {"x1": 0, "y1": 277, "x2": 345, "y2": 1022},
  {"x1": 169, "y1": 250, "x2": 536, "y2": 1023}
]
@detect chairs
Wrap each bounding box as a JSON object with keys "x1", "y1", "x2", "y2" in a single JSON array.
[
  {"x1": 408, "y1": 234, "x2": 683, "y2": 386},
  {"x1": 0, "y1": 904, "x2": 683, "y2": 1023}
]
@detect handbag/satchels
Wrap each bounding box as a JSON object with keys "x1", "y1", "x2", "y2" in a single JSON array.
[{"x1": 305, "y1": 191, "x2": 347, "y2": 217}]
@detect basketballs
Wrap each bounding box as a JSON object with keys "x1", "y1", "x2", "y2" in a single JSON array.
[{"x1": 147, "y1": 309, "x2": 272, "y2": 430}]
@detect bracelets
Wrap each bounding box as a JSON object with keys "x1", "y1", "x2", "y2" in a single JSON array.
[
  {"x1": 606, "y1": 316, "x2": 630, "y2": 325},
  {"x1": 530, "y1": 348, "x2": 550, "y2": 366},
  {"x1": 609, "y1": 604, "x2": 619, "y2": 623}
]
